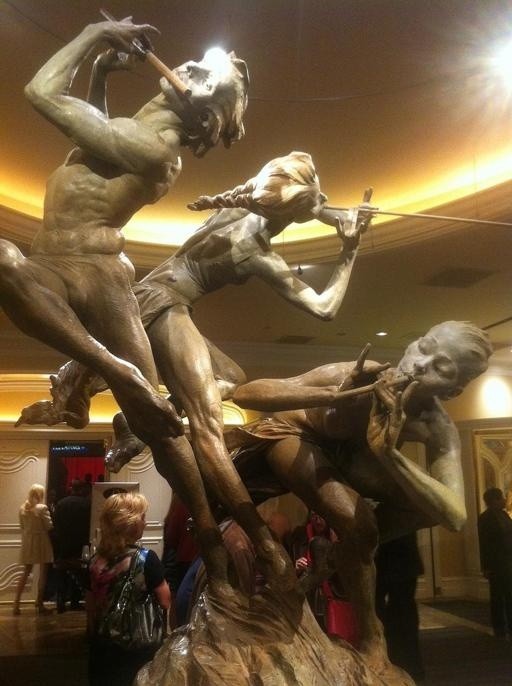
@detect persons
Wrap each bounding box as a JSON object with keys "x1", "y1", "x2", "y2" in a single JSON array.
[
  {"x1": 379, "y1": 530, "x2": 427, "y2": 686},
  {"x1": 14, "y1": 483, "x2": 91, "y2": 616},
  {"x1": 477, "y1": 488, "x2": 512, "y2": 640},
  {"x1": 161, "y1": 493, "x2": 208, "y2": 628},
  {"x1": 0, "y1": 14, "x2": 251, "y2": 602},
  {"x1": 84, "y1": 493, "x2": 171, "y2": 686},
  {"x1": 282, "y1": 508, "x2": 347, "y2": 642},
  {"x1": 15, "y1": 150, "x2": 380, "y2": 591},
  {"x1": 229, "y1": 319, "x2": 494, "y2": 672}
]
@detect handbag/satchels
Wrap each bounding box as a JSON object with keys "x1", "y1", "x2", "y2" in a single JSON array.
[
  {"x1": 96, "y1": 576, "x2": 164, "y2": 654},
  {"x1": 323, "y1": 600, "x2": 362, "y2": 644}
]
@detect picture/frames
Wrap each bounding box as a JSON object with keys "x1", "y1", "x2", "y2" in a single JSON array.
[{"x1": 474, "y1": 427, "x2": 512, "y2": 520}]
